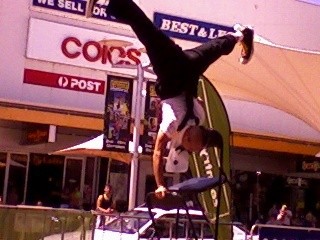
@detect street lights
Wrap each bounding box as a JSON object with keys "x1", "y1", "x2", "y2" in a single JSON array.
[{"x1": 96, "y1": 36, "x2": 145, "y2": 211}]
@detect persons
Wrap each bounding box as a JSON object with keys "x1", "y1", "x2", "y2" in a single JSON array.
[
  {"x1": 268, "y1": 203, "x2": 320, "y2": 228},
  {"x1": 96, "y1": 185, "x2": 116, "y2": 213},
  {"x1": 64, "y1": 186, "x2": 82, "y2": 208},
  {"x1": 86, "y1": 0, "x2": 254, "y2": 199}
]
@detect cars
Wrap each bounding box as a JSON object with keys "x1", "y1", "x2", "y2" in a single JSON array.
[{"x1": 38, "y1": 203, "x2": 257, "y2": 240}]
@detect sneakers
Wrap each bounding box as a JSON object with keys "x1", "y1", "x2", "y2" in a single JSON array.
[
  {"x1": 240, "y1": 26, "x2": 254, "y2": 64},
  {"x1": 85, "y1": 0, "x2": 95, "y2": 18}
]
